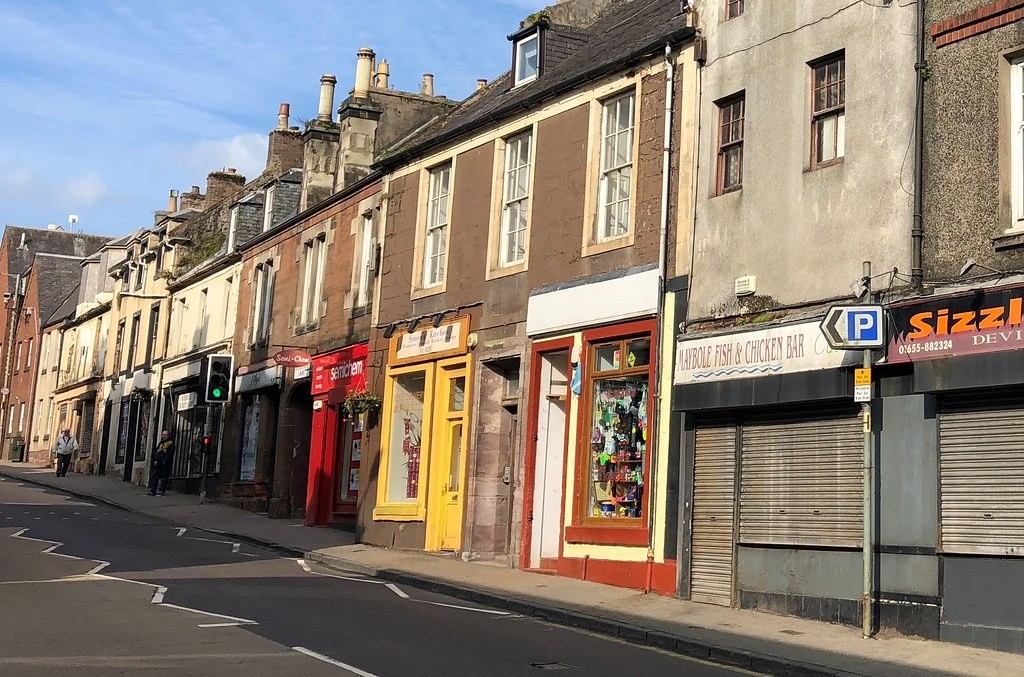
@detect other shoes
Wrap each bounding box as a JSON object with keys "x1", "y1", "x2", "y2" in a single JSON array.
[
  {"x1": 56, "y1": 472, "x2": 60, "y2": 477},
  {"x1": 143, "y1": 491, "x2": 157, "y2": 497},
  {"x1": 156, "y1": 492, "x2": 165, "y2": 496},
  {"x1": 60, "y1": 473, "x2": 65, "y2": 477}
]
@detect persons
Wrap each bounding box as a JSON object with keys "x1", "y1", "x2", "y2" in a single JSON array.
[
  {"x1": 144, "y1": 430, "x2": 175, "y2": 497},
  {"x1": 52, "y1": 429, "x2": 80, "y2": 477}
]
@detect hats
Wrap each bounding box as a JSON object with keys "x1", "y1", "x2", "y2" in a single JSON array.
[{"x1": 62, "y1": 428, "x2": 70, "y2": 431}]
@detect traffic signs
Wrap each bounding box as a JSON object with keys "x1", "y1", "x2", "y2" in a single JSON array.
[{"x1": 819, "y1": 303, "x2": 886, "y2": 350}]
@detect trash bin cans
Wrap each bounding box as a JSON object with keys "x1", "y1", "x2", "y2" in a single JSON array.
[{"x1": 8, "y1": 436, "x2": 25, "y2": 462}]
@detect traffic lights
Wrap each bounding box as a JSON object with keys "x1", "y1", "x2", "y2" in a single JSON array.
[
  {"x1": 200, "y1": 436, "x2": 212, "y2": 453},
  {"x1": 204, "y1": 354, "x2": 235, "y2": 403}
]
